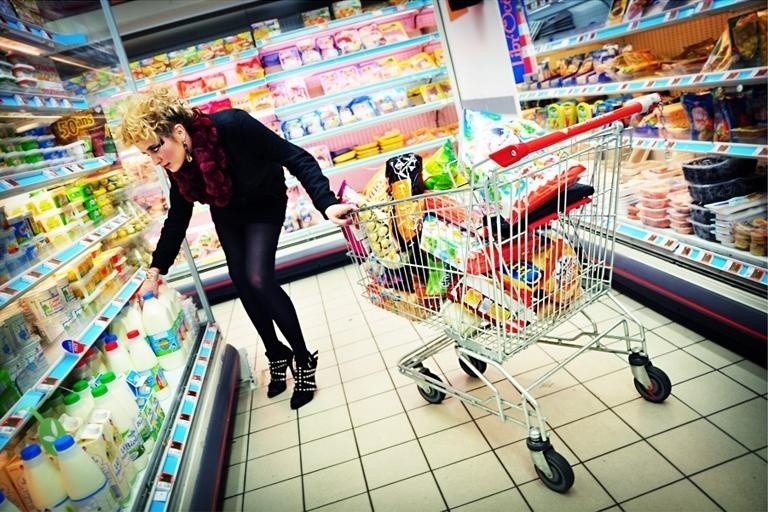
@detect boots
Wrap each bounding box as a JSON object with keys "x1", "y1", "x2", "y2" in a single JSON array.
[
  {"x1": 290, "y1": 350, "x2": 316, "y2": 409},
  {"x1": 265, "y1": 342, "x2": 295, "y2": 397}
]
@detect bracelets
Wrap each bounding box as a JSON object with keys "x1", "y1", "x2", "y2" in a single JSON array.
[{"x1": 145, "y1": 269, "x2": 158, "y2": 280}]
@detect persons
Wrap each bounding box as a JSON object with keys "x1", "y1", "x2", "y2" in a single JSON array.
[{"x1": 122, "y1": 87, "x2": 360, "y2": 408}]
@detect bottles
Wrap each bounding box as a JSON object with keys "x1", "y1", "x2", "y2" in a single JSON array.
[{"x1": 21, "y1": 435, "x2": 121, "y2": 512}]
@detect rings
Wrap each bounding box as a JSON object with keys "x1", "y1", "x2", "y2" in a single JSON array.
[{"x1": 136, "y1": 297, "x2": 143, "y2": 303}]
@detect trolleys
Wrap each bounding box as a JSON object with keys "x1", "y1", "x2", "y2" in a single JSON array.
[{"x1": 338, "y1": 92, "x2": 672, "y2": 492}]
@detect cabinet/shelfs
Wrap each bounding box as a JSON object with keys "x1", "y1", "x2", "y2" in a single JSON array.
[
  {"x1": 493, "y1": 0, "x2": 768, "y2": 345},
  {"x1": 0, "y1": 0, "x2": 240, "y2": 512},
  {"x1": 45, "y1": 0, "x2": 472, "y2": 309}
]
